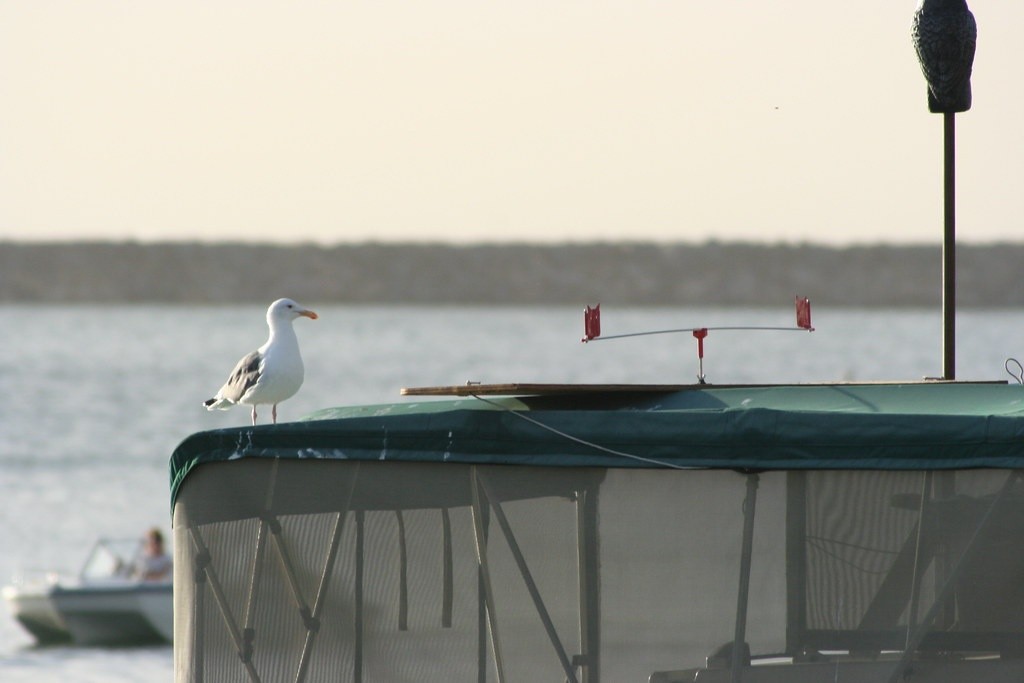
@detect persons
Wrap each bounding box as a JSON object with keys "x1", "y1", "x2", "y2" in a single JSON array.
[{"x1": 116, "y1": 529, "x2": 174, "y2": 583}]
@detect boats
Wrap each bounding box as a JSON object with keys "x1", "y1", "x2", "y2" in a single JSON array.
[
  {"x1": 11, "y1": 589, "x2": 73, "y2": 649},
  {"x1": 46, "y1": 571, "x2": 175, "y2": 648}
]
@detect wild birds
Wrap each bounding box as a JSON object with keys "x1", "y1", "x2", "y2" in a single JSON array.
[{"x1": 202, "y1": 297, "x2": 317, "y2": 424}]
